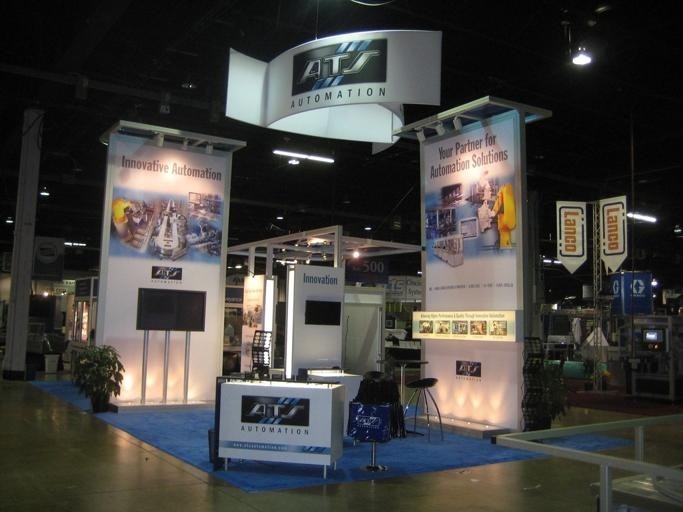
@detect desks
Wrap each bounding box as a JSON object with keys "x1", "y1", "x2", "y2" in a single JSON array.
[
  {"x1": 489, "y1": 411, "x2": 682, "y2": 512},
  {"x1": 376, "y1": 358, "x2": 429, "y2": 436}
]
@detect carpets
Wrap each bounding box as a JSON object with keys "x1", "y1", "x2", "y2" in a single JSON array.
[
  {"x1": 562, "y1": 383, "x2": 682, "y2": 416},
  {"x1": 31, "y1": 377, "x2": 647, "y2": 491}
]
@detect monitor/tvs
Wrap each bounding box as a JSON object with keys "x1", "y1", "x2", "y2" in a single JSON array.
[
  {"x1": 135, "y1": 286, "x2": 207, "y2": 332},
  {"x1": 641, "y1": 329, "x2": 663, "y2": 342},
  {"x1": 305, "y1": 300, "x2": 341, "y2": 325}
]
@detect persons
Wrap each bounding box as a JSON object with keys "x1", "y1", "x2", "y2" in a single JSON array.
[{"x1": 223, "y1": 315, "x2": 235, "y2": 338}]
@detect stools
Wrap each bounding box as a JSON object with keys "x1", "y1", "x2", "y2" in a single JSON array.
[{"x1": 402, "y1": 377, "x2": 443, "y2": 439}]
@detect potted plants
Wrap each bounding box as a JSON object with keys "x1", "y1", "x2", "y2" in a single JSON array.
[{"x1": 71, "y1": 343, "x2": 125, "y2": 412}]
